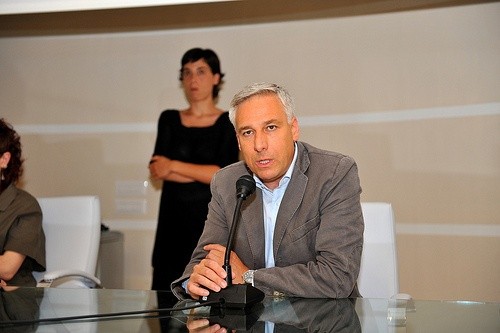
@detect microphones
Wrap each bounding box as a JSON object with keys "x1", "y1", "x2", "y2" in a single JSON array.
[{"x1": 199, "y1": 174, "x2": 265, "y2": 305}]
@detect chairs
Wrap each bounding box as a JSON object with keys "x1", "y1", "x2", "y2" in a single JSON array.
[
  {"x1": 32, "y1": 196, "x2": 102, "y2": 289},
  {"x1": 356, "y1": 203, "x2": 398, "y2": 301}
]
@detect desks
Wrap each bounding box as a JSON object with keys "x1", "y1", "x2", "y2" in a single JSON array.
[{"x1": 0, "y1": 285, "x2": 500, "y2": 333}]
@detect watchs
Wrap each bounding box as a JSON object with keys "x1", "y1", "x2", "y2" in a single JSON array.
[{"x1": 244, "y1": 269, "x2": 257, "y2": 287}]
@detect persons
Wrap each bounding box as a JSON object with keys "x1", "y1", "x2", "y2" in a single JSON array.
[
  {"x1": 0, "y1": 287, "x2": 45, "y2": 333},
  {"x1": 0, "y1": 117, "x2": 47, "y2": 287},
  {"x1": 170, "y1": 82, "x2": 366, "y2": 303},
  {"x1": 156, "y1": 291, "x2": 363, "y2": 333},
  {"x1": 149, "y1": 48, "x2": 240, "y2": 291}
]
